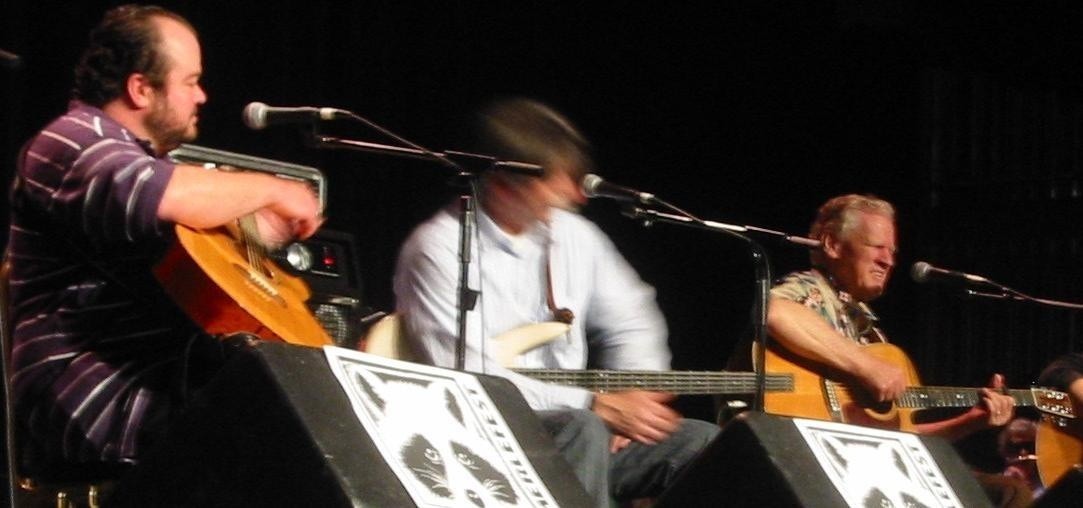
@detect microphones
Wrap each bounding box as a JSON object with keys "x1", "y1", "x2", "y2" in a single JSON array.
[
  {"x1": 910, "y1": 261, "x2": 991, "y2": 286},
  {"x1": 242, "y1": 101, "x2": 352, "y2": 130},
  {"x1": 579, "y1": 173, "x2": 654, "y2": 206}
]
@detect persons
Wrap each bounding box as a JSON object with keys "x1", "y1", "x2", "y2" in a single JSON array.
[
  {"x1": 3, "y1": 4, "x2": 322, "y2": 484},
  {"x1": 751, "y1": 193, "x2": 1033, "y2": 508},
  {"x1": 1036, "y1": 352, "x2": 1083, "y2": 402},
  {"x1": 394, "y1": 98, "x2": 723, "y2": 508}
]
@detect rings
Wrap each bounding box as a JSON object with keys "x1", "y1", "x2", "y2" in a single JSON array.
[{"x1": 996, "y1": 410, "x2": 1001, "y2": 416}]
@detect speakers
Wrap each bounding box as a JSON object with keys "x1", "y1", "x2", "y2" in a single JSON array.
[
  {"x1": 649, "y1": 410, "x2": 994, "y2": 508},
  {"x1": 102, "y1": 340, "x2": 599, "y2": 508}
]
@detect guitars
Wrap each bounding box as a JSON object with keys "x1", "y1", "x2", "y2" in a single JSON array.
[
  {"x1": 154, "y1": 163, "x2": 335, "y2": 348},
  {"x1": 356, "y1": 313, "x2": 794, "y2": 394},
  {"x1": 749, "y1": 342, "x2": 1077, "y2": 438}
]
